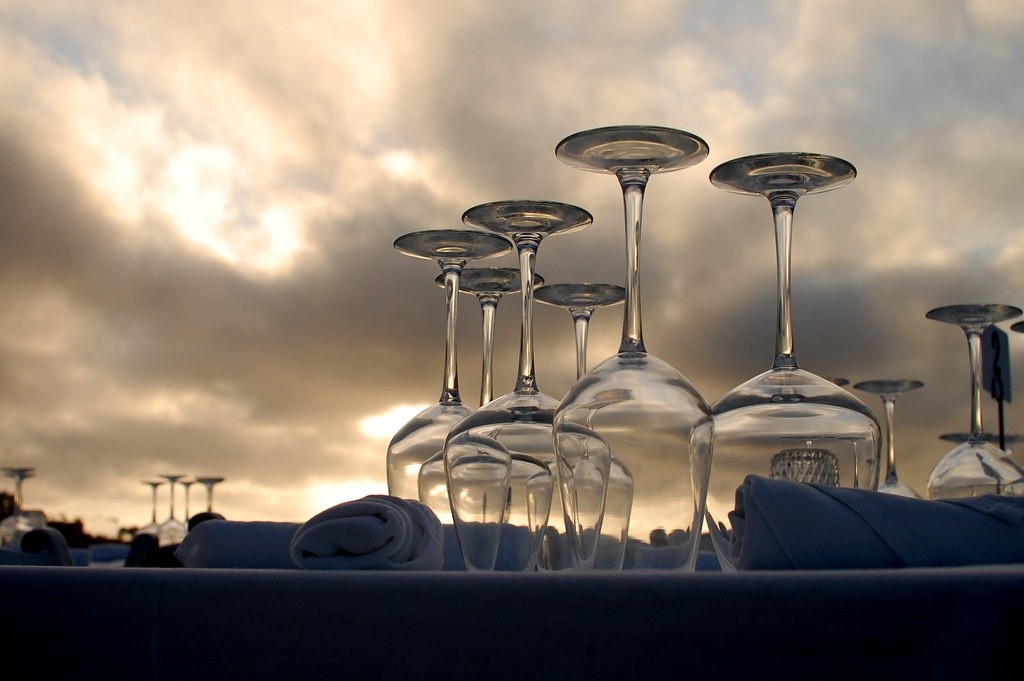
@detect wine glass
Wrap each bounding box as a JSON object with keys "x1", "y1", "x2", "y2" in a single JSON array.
[
  {"x1": 534, "y1": 283, "x2": 625, "y2": 381},
  {"x1": 690, "y1": 152, "x2": 882, "y2": 571},
  {"x1": 155, "y1": 473, "x2": 189, "y2": 567},
  {"x1": 0, "y1": 466, "x2": 36, "y2": 553},
  {"x1": 188, "y1": 478, "x2": 226, "y2": 532},
  {"x1": 853, "y1": 379, "x2": 925, "y2": 500},
  {"x1": 553, "y1": 125, "x2": 713, "y2": 575},
  {"x1": 386, "y1": 229, "x2": 513, "y2": 572},
  {"x1": 177, "y1": 480, "x2": 198, "y2": 531},
  {"x1": 926, "y1": 304, "x2": 1024, "y2": 502},
  {"x1": 418, "y1": 267, "x2": 544, "y2": 572},
  {"x1": 124, "y1": 482, "x2": 169, "y2": 567},
  {"x1": 443, "y1": 199, "x2": 594, "y2": 574}
]
